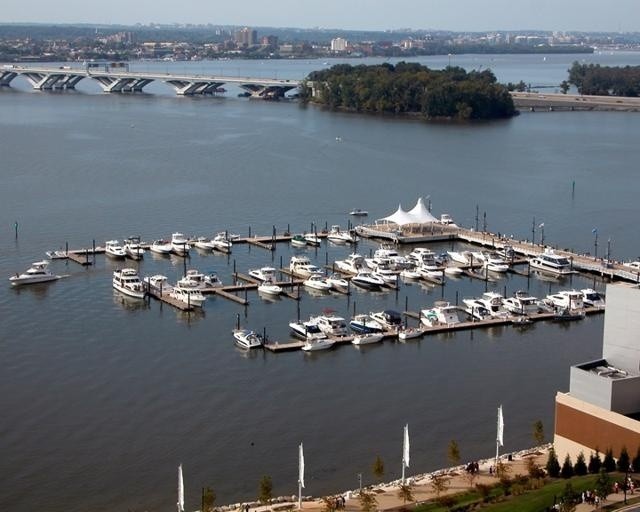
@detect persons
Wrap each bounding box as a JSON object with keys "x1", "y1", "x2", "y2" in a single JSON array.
[
  {"x1": 340, "y1": 495, "x2": 346, "y2": 508},
  {"x1": 581, "y1": 488, "x2": 599, "y2": 508},
  {"x1": 466, "y1": 460, "x2": 475, "y2": 472},
  {"x1": 474, "y1": 460, "x2": 478, "y2": 467},
  {"x1": 245, "y1": 503, "x2": 250, "y2": 512},
  {"x1": 630, "y1": 479, "x2": 635, "y2": 495},
  {"x1": 615, "y1": 480, "x2": 619, "y2": 493},
  {"x1": 335, "y1": 497, "x2": 339, "y2": 510},
  {"x1": 489, "y1": 464, "x2": 493, "y2": 475}
]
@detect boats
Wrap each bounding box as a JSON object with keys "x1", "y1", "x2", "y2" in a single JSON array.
[
  {"x1": 105, "y1": 231, "x2": 240, "y2": 260},
  {"x1": 8, "y1": 262, "x2": 59, "y2": 286},
  {"x1": 349, "y1": 209, "x2": 370, "y2": 216},
  {"x1": 291, "y1": 225, "x2": 360, "y2": 248},
  {"x1": 232, "y1": 328, "x2": 264, "y2": 350},
  {"x1": 623, "y1": 261, "x2": 640, "y2": 270},
  {"x1": 112, "y1": 268, "x2": 223, "y2": 311},
  {"x1": 529, "y1": 253, "x2": 580, "y2": 275},
  {"x1": 247, "y1": 247, "x2": 515, "y2": 295}
]
